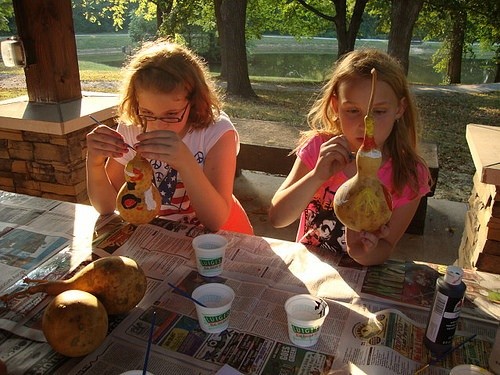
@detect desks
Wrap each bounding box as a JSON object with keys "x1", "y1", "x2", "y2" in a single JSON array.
[{"x1": 0, "y1": 186, "x2": 500, "y2": 375}]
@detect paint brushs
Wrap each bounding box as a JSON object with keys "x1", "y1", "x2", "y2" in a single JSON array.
[
  {"x1": 414, "y1": 334, "x2": 476, "y2": 375},
  {"x1": 89, "y1": 115, "x2": 137, "y2": 152},
  {"x1": 175, "y1": 288, "x2": 206, "y2": 307},
  {"x1": 142, "y1": 312, "x2": 156, "y2": 375}
]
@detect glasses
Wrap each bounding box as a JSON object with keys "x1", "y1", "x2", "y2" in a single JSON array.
[{"x1": 135, "y1": 92, "x2": 196, "y2": 123}]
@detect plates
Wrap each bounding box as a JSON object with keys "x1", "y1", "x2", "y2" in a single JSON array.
[{"x1": 449, "y1": 364, "x2": 496, "y2": 375}]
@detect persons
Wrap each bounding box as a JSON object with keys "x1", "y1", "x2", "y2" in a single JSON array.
[
  {"x1": 85, "y1": 39, "x2": 255, "y2": 237},
  {"x1": 269, "y1": 47, "x2": 433, "y2": 266}
]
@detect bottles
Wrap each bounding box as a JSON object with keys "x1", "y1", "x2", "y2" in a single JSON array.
[{"x1": 421, "y1": 265, "x2": 468, "y2": 355}]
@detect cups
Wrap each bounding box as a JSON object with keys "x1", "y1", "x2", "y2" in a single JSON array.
[
  {"x1": 119, "y1": 369, "x2": 155, "y2": 375},
  {"x1": 488, "y1": 326, "x2": 500, "y2": 374},
  {"x1": 192, "y1": 234, "x2": 227, "y2": 277},
  {"x1": 284, "y1": 295, "x2": 329, "y2": 347},
  {"x1": 191, "y1": 283, "x2": 235, "y2": 333}
]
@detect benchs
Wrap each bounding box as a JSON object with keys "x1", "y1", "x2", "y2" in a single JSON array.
[{"x1": 230, "y1": 115, "x2": 440, "y2": 236}]
